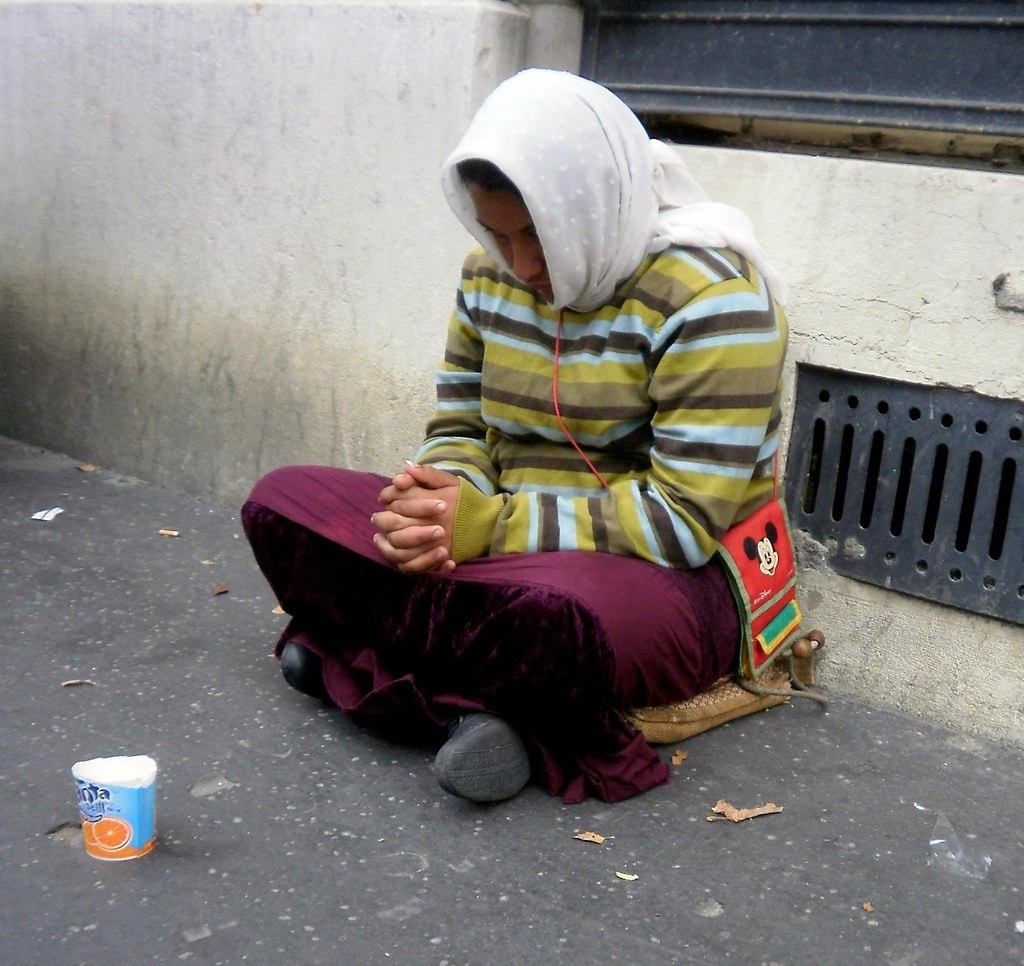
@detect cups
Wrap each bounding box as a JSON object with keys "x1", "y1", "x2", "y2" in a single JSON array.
[{"x1": 72, "y1": 755, "x2": 158, "y2": 861}]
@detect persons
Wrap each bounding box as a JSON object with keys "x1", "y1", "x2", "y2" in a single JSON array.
[{"x1": 240, "y1": 66, "x2": 799, "y2": 806}]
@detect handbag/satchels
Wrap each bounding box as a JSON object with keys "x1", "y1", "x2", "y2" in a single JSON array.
[{"x1": 715, "y1": 498, "x2": 803, "y2": 678}]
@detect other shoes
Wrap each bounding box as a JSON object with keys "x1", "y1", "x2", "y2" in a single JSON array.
[
  {"x1": 434, "y1": 712, "x2": 531, "y2": 800},
  {"x1": 280, "y1": 643, "x2": 330, "y2": 700}
]
